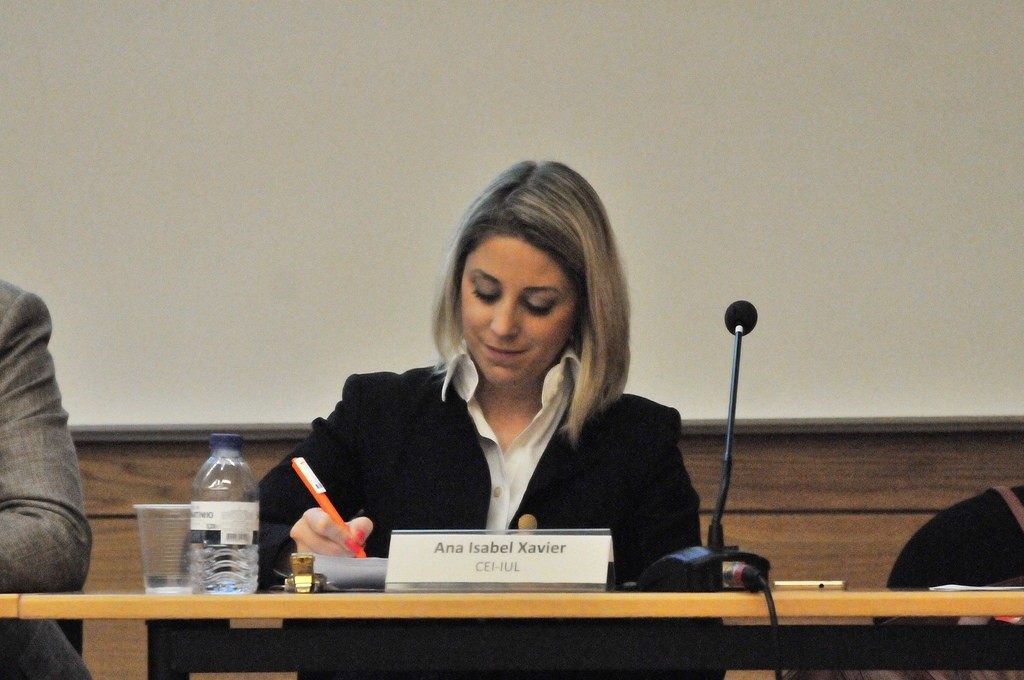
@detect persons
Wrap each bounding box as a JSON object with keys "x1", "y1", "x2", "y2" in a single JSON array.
[
  {"x1": 0, "y1": 279, "x2": 93, "y2": 680},
  {"x1": 180, "y1": 160, "x2": 701, "y2": 591}
]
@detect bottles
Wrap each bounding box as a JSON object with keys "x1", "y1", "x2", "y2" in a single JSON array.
[{"x1": 189, "y1": 433, "x2": 260, "y2": 595}]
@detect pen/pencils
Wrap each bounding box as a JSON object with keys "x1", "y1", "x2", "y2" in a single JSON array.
[{"x1": 290, "y1": 455, "x2": 366, "y2": 559}]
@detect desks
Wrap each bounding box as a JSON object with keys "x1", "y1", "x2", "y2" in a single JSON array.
[{"x1": 0, "y1": 587, "x2": 1024, "y2": 680}]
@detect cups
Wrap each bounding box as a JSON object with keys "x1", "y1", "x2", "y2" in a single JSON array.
[{"x1": 133, "y1": 503, "x2": 191, "y2": 594}]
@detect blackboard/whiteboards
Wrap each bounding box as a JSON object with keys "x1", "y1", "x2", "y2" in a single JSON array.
[{"x1": 0, "y1": 3, "x2": 1024, "y2": 437}]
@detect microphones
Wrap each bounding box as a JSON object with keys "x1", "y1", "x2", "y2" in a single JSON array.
[{"x1": 638, "y1": 301, "x2": 771, "y2": 596}]
